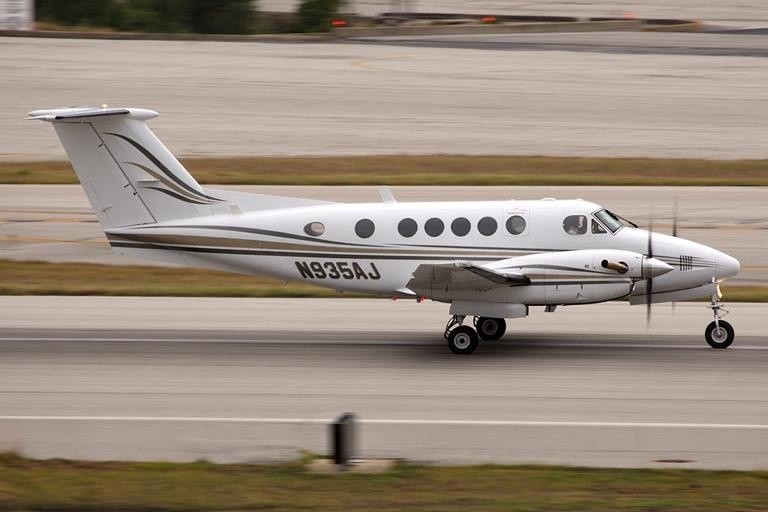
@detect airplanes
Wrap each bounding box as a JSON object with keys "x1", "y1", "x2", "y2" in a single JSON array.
[{"x1": 27, "y1": 106, "x2": 739, "y2": 354}]
